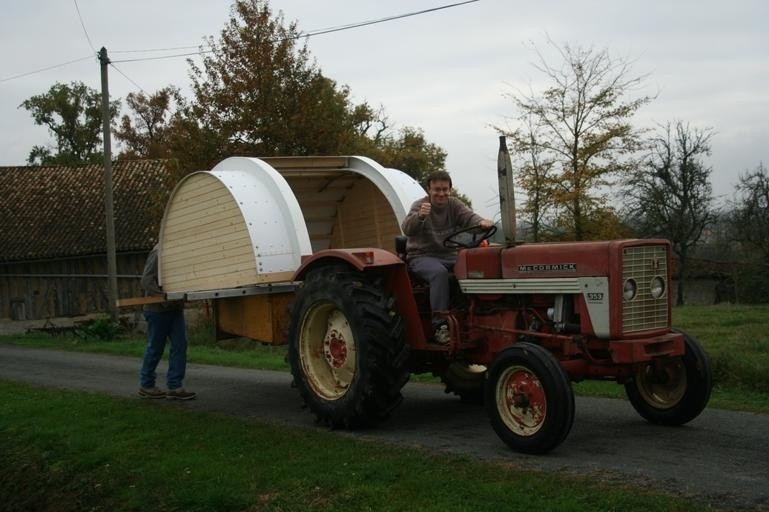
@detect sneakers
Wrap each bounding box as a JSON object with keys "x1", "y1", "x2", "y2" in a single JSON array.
[
  {"x1": 433, "y1": 324, "x2": 453, "y2": 345},
  {"x1": 166, "y1": 388, "x2": 198, "y2": 403},
  {"x1": 138, "y1": 383, "x2": 164, "y2": 399}
]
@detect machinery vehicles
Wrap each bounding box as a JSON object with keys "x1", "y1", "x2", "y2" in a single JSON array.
[{"x1": 281, "y1": 134, "x2": 712, "y2": 456}]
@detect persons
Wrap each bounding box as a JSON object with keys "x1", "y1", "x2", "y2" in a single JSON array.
[
  {"x1": 403, "y1": 170, "x2": 494, "y2": 344},
  {"x1": 135, "y1": 241, "x2": 198, "y2": 402}
]
